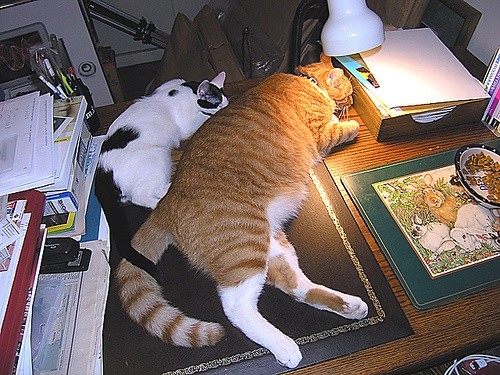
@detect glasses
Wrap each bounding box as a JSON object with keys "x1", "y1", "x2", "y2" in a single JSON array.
[{"x1": 319, "y1": 1, "x2": 386, "y2": 58}]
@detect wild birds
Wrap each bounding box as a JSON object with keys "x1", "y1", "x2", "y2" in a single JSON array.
[{"x1": 0, "y1": 22, "x2": 50, "y2": 103}]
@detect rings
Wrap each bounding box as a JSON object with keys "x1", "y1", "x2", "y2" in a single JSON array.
[{"x1": 38, "y1": 58, "x2": 82, "y2": 98}]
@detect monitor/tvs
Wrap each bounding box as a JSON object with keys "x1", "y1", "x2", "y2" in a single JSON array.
[
  {"x1": 479, "y1": 45, "x2": 500, "y2": 139},
  {"x1": 0, "y1": 89, "x2": 111, "y2": 238}
]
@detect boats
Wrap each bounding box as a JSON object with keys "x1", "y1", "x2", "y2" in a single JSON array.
[{"x1": 78, "y1": 60, "x2": 96, "y2": 77}]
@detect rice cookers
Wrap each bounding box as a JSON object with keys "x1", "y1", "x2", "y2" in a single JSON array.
[{"x1": 41, "y1": 237, "x2": 91, "y2": 272}]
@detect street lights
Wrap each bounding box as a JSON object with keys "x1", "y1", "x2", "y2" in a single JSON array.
[
  {"x1": 154, "y1": 13, "x2": 218, "y2": 85},
  {"x1": 193, "y1": 4, "x2": 246, "y2": 84}
]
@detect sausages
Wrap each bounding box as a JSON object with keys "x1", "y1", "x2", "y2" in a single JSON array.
[
  {"x1": 99, "y1": 71, "x2": 229, "y2": 209},
  {"x1": 114, "y1": 52, "x2": 368, "y2": 368}
]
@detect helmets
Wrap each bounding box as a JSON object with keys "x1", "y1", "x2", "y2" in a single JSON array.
[{"x1": 454, "y1": 143, "x2": 500, "y2": 210}]
